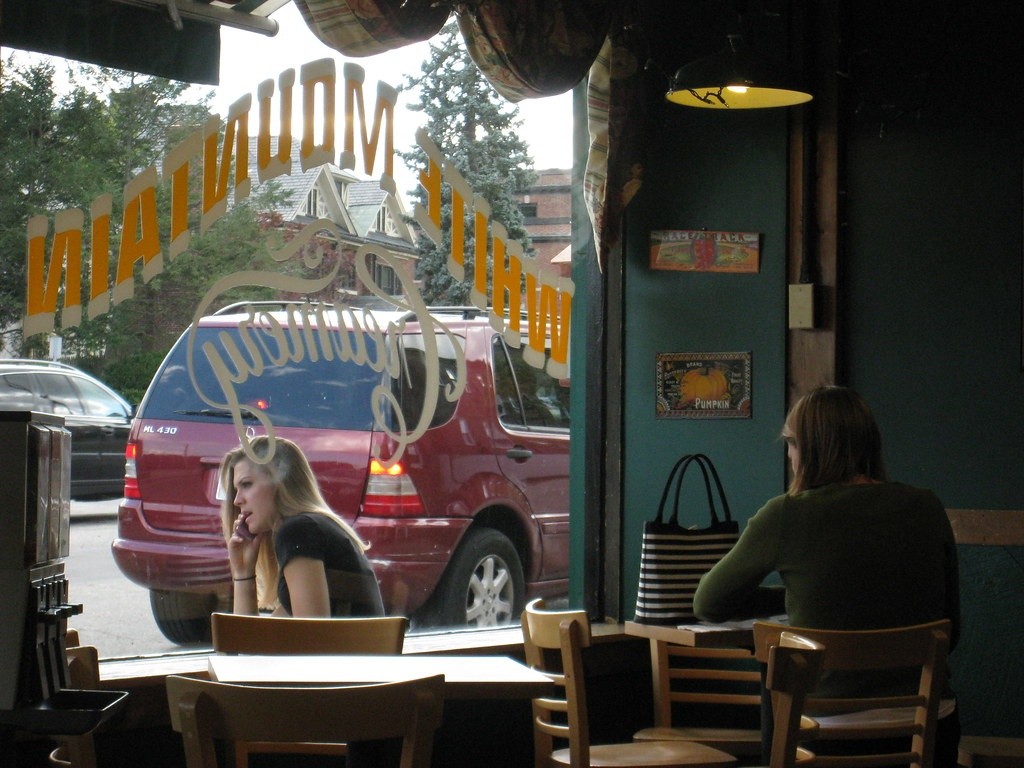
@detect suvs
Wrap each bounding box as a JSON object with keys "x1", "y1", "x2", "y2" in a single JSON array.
[
  {"x1": 110, "y1": 299, "x2": 571, "y2": 647},
  {"x1": 0, "y1": 357, "x2": 143, "y2": 502}
]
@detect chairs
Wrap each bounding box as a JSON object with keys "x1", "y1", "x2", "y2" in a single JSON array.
[
  {"x1": 161, "y1": 674, "x2": 450, "y2": 768},
  {"x1": 752, "y1": 618, "x2": 952, "y2": 768},
  {"x1": 521, "y1": 597, "x2": 764, "y2": 768},
  {"x1": 211, "y1": 611, "x2": 410, "y2": 768}
]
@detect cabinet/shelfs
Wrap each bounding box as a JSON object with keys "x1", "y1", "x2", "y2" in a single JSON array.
[{"x1": 656, "y1": 350, "x2": 753, "y2": 420}]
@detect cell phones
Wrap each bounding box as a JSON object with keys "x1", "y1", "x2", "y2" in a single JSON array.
[{"x1": 235, "y1": 520, "x2": 258, "y2": 541}]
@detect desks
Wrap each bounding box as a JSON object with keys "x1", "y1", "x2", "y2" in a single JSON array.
[
  {"x1": 208, "y1": 655, "x2": 555, "y2": 701},
  {"x1": 621, "y1": 620, "x2": 790, "y2": 728}
]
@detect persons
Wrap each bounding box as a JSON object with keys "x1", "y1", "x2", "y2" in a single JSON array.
[
  {"x1": 694, "y1": 386, "x2": 961, "y2": 768},
  {"x1": 219, "y1": 436, "x2": 387, "y2": 619}
]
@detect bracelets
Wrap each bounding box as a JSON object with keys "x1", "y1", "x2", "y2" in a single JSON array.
[{"x1": 234, "y1": 575, "x2": 256, "y2": 581}]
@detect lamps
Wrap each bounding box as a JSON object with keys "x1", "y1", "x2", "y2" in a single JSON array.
[{"x1": 664, "y1": 32, "x2": 814, "y2": 110}]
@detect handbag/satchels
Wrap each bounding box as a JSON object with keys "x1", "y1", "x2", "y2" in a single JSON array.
[{"x1": 633, "y1": 452, "x2": 741, "y2": 624}]
